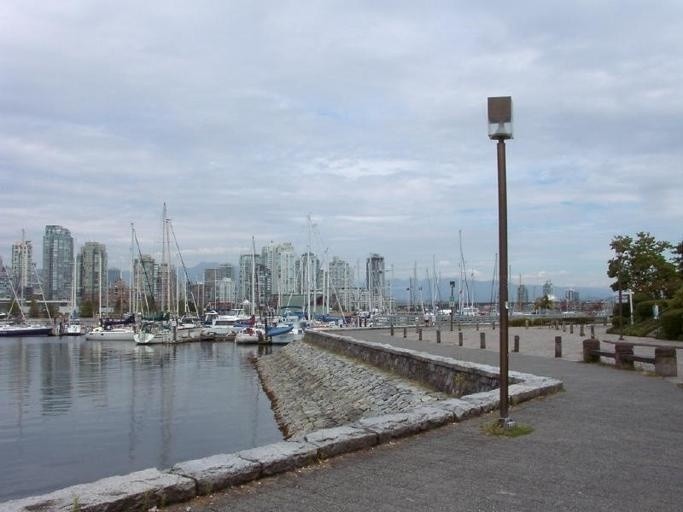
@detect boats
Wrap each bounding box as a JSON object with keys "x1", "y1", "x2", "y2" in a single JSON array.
[{"x1": 52, "y1": 318, "x2": 82, "y2": 336}]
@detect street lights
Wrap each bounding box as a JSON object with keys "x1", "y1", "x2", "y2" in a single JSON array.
[{"x1": 486, "y1": 95, "x2": 514, "y2": 431}]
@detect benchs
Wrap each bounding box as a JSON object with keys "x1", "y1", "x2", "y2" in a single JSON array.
[{"x1": 604, "y1": 338, "x2": 683, "y2": 376}]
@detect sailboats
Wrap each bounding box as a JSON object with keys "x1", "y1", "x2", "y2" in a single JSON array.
[
  {"x1": 0, "y1": 228, "x2": 55, "y2": 335},
  {"x1": 85, "y1": 197, "x2": 483, "y2": 350}
]
